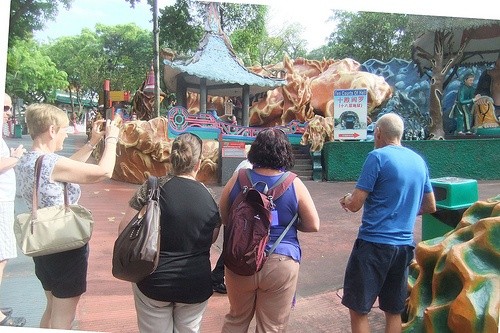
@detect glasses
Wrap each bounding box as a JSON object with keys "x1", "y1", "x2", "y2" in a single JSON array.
[
  {"x1": 176, "y1": 131, "x2": 203, "y2": 144},
  {"x1": 4, "y1": 105, "x2": 13, "y2": 113}
]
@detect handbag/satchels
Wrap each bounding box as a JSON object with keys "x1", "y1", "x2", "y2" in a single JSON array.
[
  {"x1": 112, "y1": 177, "x2": 160, "y2": 283},
  {"x1": 15, "y1": 155, "x2": 95, "y2": 256}
]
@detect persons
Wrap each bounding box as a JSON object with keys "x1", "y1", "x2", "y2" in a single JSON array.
[
  {"x1": 212, "y1": 127, "x2": 320, "y2": 333},
  {"x1": 15, "y1": 102, "x2": 122, "y2": 330},
  {"x1": 117, "y1": 132, "x2": 221, "y2": 333},
  {"x1": 454, "y1": 73, "x2": 482, "y2": 136},
  {"x1": 339, "y1": 112, "x2": 437, "y2": 333},
  {"x1": 0, "y1": 92, "x2": 27, "y2": 327}
]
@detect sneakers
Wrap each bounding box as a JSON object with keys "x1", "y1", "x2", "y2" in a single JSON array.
[{"x1": 212, "y1": 280, "x2": 227, "y2": 294}]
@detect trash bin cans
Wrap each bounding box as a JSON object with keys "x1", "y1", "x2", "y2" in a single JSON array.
[
  {"x1": 408, "y1": 176, "x2": 479, "y2": 287},
  {"x1": 14, "y1": 124, "x2": 22, "y2": 138}
]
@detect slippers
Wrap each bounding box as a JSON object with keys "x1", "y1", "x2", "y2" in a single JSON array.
[
  {"x1": 0, "y1": 316, "x2": 26, "y2": 327},
  {"x1": 0, "y1": 307, "x2": 13, "y2": 315}
]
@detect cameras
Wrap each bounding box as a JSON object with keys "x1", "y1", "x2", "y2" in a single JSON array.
[{"x1": 102, "y1": 121, "x2": 106, "y2": 131}]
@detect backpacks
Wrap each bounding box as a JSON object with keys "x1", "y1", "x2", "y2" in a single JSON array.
[{"x1": 220, "y1": 168, "x2": 298, "y2": 274}]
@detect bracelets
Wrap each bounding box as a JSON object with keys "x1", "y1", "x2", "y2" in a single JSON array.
[
  {"x1": 104, "y1": 135, "x2": 118, "y2": 141},
  {"x1": 87, "y1": 139, "x2": 96, "y2": 151}
]
